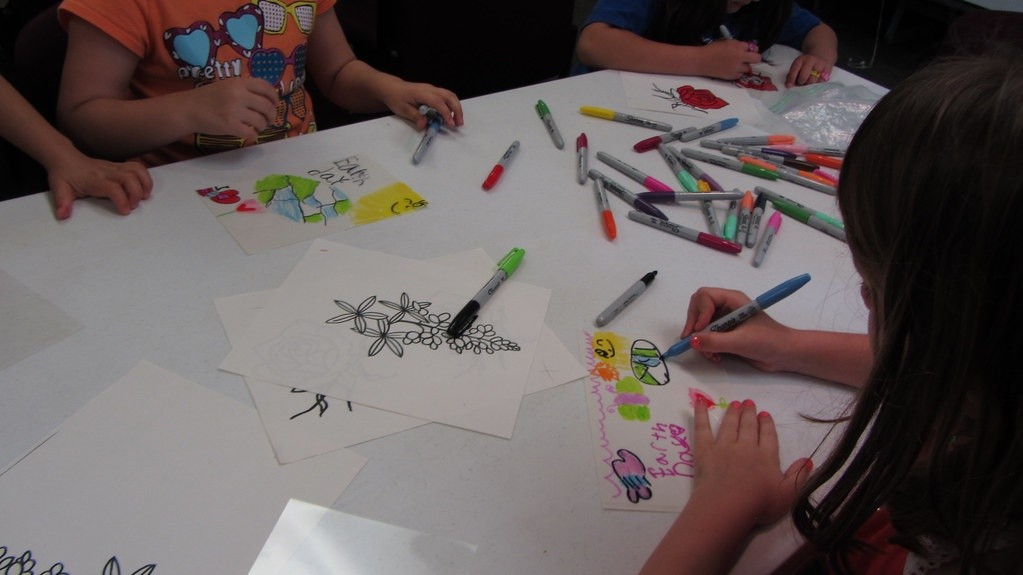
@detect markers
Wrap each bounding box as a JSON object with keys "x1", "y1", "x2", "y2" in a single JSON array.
[{"x1": 578, "y1": 106, "x2": 671, "y2": 130}]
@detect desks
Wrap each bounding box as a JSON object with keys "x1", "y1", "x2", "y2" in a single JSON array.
[{"x1": 0, "y1": 44, "x2": 897, "y2": 575}]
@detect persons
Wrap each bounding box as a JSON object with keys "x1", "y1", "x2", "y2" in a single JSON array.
[
  {"x1": 57, "y1": 0, "x2": 464, "y2": 169},
  {"x1": 637, "y1": 55, "x2": 1023, "y2": 575},
  {"x1": 0, "y1": 76, "x2": 153, "y2": 221},
  {"x1": 569, "y1": 0, "x2": 837, "y2": 88}
]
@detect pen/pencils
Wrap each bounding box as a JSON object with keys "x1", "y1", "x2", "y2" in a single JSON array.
[
  {"x1": 660, "y1": 272, "x2": 811, "y2": 360},
  {"x1": 594, "y1": 180, "x2": 619, "y2": 240},
  {"x1": 482, "y1": 141, "x2": 520, "y2": 191},
  {"x1": 721, "y1": 25, "x2": 739, "y2": 42},
  {"x1": 536, "y1": 97, "x2": 565, "y2": 150},
  {"x1": 576, "y1": 131, "x2": 588, "y2": 185},
  {"x1": 445, "y1": 248, "x2": 525, "y2": 338},
  {"x1": 626, "y1": 211, "x2": 741, "y2": 257},
  {"x1": 412, "y1": 113, "x2": 442, "y2": 165},
  {"x1": 590, "y1": 169, "x2": 669, "y2": 225},
  {"x1": 597, "y1": 269, "x2": 657, "y2": 331},
  {"x1": 635, "y1": 117, "x2": 850, "y2": 267},
  {"x1": 596, "y1": 151, "x2": 674, "y2": 197}
]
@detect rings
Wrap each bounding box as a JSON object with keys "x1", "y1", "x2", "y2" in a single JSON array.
[
  {"x1": 822, "y1": 73, "x2": 829, "y2": 81},
  {"x1": 747, "y1": 42, "x2": 755, "y2": 52},
  {"x1": 811, "y1": 70, "x2": 820, "y2": 78}
]
